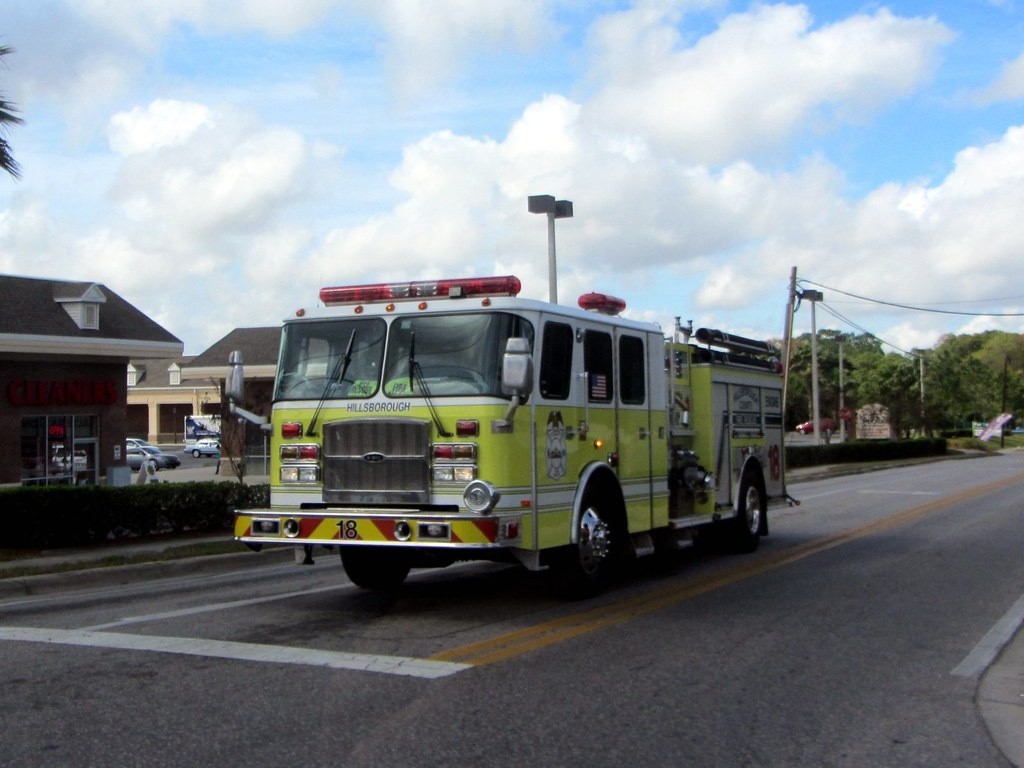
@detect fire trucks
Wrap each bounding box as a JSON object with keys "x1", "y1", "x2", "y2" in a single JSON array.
[{"x1": 233, "y1": 275, "x2": 806, "y2": 602}]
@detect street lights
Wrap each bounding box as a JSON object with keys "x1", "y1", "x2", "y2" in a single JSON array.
[
  {"x1": 835, "y1": 336, "x2": 846, "y2": 443},
  {"x1": 527, "y1": 195, "x2": 574, "y2": 304},
  {"x1": 917, "y1": 349, "x2": 926, "y2": 416},
  {"x1": 802, "y1": 290, "x2": 823, "y2": 444}
]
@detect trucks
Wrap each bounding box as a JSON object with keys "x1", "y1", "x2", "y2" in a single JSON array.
[{"x1": 183, "y1": 416, "x2": 222, "y2": 445}]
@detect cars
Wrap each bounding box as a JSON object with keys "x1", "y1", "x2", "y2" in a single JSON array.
[
  {"x1": 115, "y1": 438, "x2": 159, "y2": 454},
  {"x1": 796, "y1": 418, "x2": 838, "y2": 434},
  {"x1": 50, "y1": 445, "x2": 88, "y2": 469},
  {"x1": 184, "y1": 441, "x2": 221, "y2": 459},
  {"x1": 125, "y1": 447, "x2": 182, "y2": 472}
]
